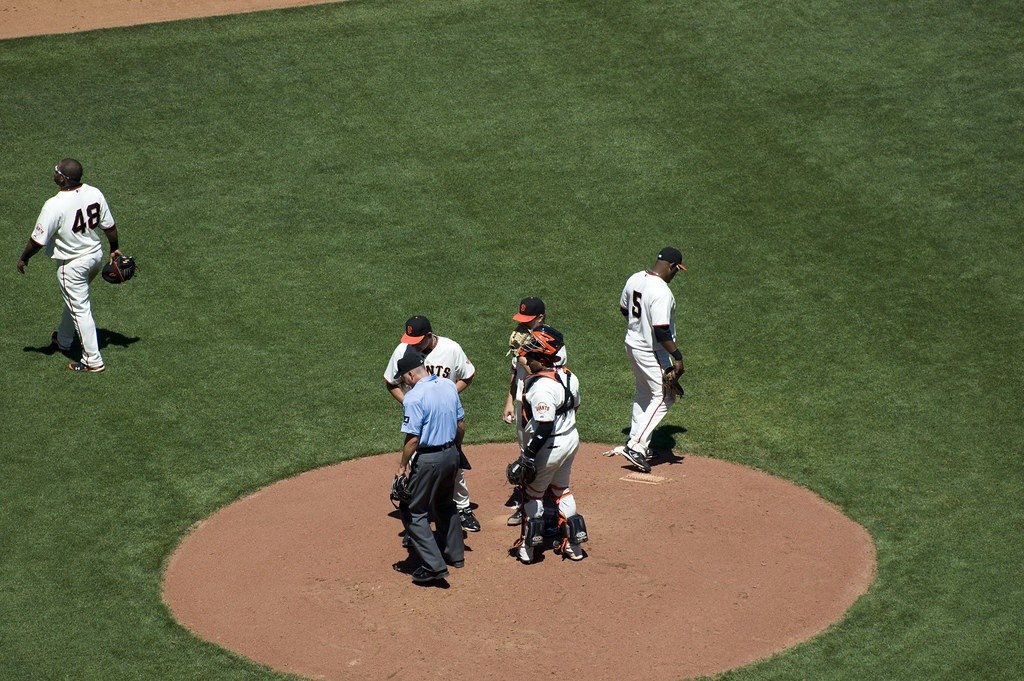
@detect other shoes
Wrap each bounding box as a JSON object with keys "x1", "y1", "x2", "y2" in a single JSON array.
[
  {"x1": 518, "y1": 547, "x2": 534, "y2": 562},
  {"x1": 565, "y1": 545, "x2": 584, "y2": 560}
]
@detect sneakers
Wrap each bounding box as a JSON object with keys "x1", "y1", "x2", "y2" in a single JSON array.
[
  {"x1": 646, "y1": 448, "x2": 660, "y2": 459},
  {"x1": 507, "y1": 511, "x2": 522, "y2": 525},
  {"x1": 621, "y1": 445, "x2": 650, "y2": 472},
  {"x1": 458, "y1": 508, "x2": 480, "y2": 532},
  {"x1": 51, "y1": 331, "x2": 70, "y2": 350},
  {"x1": 403, "y1": 533, "x2": 413, "y2": 546},
  {"x1": 69, "y1": 362, "x2": 104, "y2": 373}
]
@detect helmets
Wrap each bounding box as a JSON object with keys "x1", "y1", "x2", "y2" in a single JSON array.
[{"x1": 518, "y1": 325, "x2": 567, "y2": 366}]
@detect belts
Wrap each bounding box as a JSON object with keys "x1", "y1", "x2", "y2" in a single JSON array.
[
  {"x1": 418, "y1": 441, "x2": 454, "y2": 453},
  {"x1": 549, "y1": 434, "x2": 561, "y2": 437}
]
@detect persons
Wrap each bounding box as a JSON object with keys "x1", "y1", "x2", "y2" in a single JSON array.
[
  {"x1": 16, "y1": 159, "x2": 124, "y2": 373},
  {"x1": 389, "y1": 355, "x2": 466, "y2": 580},
  {"x1": 505, "y1": 325, "x2": 588, "y2": 562},
  {"x1": 502, "y1": 296, "x2": 567, "y2": 527},
  {"x1": 383, "y1": 316, "x2": 480, "y2": 532},
  {"x1": 619, "y1": 247, "x2": 687, "y2": 474}
]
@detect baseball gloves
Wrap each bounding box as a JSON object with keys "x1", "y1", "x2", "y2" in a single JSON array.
[
  {"x1": 390, "y1": 475, "x2": 412, "y2": 503},
  {"x1": 663, "y1": 367, "x2": 685, "y2": 397},
  {"x1": 506, "y1": 454, "x2": 536, "y2": 484},
  {"x1": 508, "y1": 324, "x2": 530, "y2": 351},
  {"x1": 101, "y1": 256, "x2": 137, "y2": 285}
]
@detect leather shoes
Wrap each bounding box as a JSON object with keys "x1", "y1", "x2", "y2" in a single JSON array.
[
  {"x1": 445, "y1": 560, "x2": 464, "y2": 567},
  {"x1": 413, "y1": 568, "x2": 448, "y2": 582}
]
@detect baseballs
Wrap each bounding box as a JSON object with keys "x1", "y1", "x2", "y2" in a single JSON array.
[{"x1": 507, "y1": 414, "x2": 516, "y2": 421}]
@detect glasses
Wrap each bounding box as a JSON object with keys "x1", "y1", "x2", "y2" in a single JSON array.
[{"x1": 54, "y1": 166, "x2": 70, "y2": 179}]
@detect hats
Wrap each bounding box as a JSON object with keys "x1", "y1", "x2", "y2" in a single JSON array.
[
  {"x1": 513, "y1": 297, "x2": 545, "y2": 322},
  {"x1": 102, "y1": 260, "x2": 124, "y2": 284},
  {"x1": 658, "y1": 247, "x2": 687, "y2": 270},
  {"x1": 400, "y1": 316, "x2": 431, "y2": 344},
  {"x1": 393, "y1": 354, "x2": 424, "y2": 379}
]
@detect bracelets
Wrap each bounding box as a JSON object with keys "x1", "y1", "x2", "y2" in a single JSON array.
[
  {"x1": 650, "y1": 270, "x2": 659, "y2": 276},
  {"x1": 670, "y1": 349, "x2": 682, "y2": 360}
]
